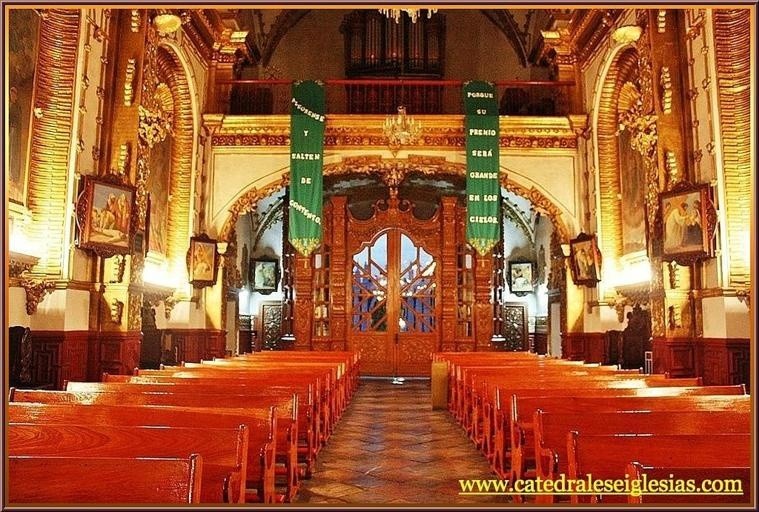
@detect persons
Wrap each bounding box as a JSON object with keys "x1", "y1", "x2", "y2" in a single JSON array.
[
  {"x1": 663, "y1": 200, "x2": 702, "y2": 252},
  {"x1": 512, "y1": 265, "x2": 531, "y2": 285},
  {"x1": 573, "y1": 245, "x2": 596, "y2": 279},
  {"x1": 91, "y1": 192, "x2": 131, "y2": 244},
  {"x1": 193, "y1": 246, "x2": 210, "y2": 275}
]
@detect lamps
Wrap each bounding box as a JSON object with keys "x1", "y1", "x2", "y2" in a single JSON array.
[
  {"x1": 378, "y1": 9, "x2": 438, "y2": 25},
  {"x1": 383, "y1": 105, "x2": 423, "y2": 144}
]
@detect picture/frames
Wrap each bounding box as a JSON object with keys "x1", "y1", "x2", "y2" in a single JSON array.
[
  {"x1": 569, "y1": 233, "x2": 601, "y2": 288},
  {"x1": 654, "y1": 180, "x2": 718, "y2": 267},
  {"x1": 75, "y1": 174, "x2": 140, "y2": 259},
  {"x1": 249, "y1": 255, "x2": 282, "y2": 295},
  {"x1": 186, "y1": 233, "x2": 220, "y2": 289},
  {"x1": 506, "y1": 257, "x2": 537, "y2": 297}
]
